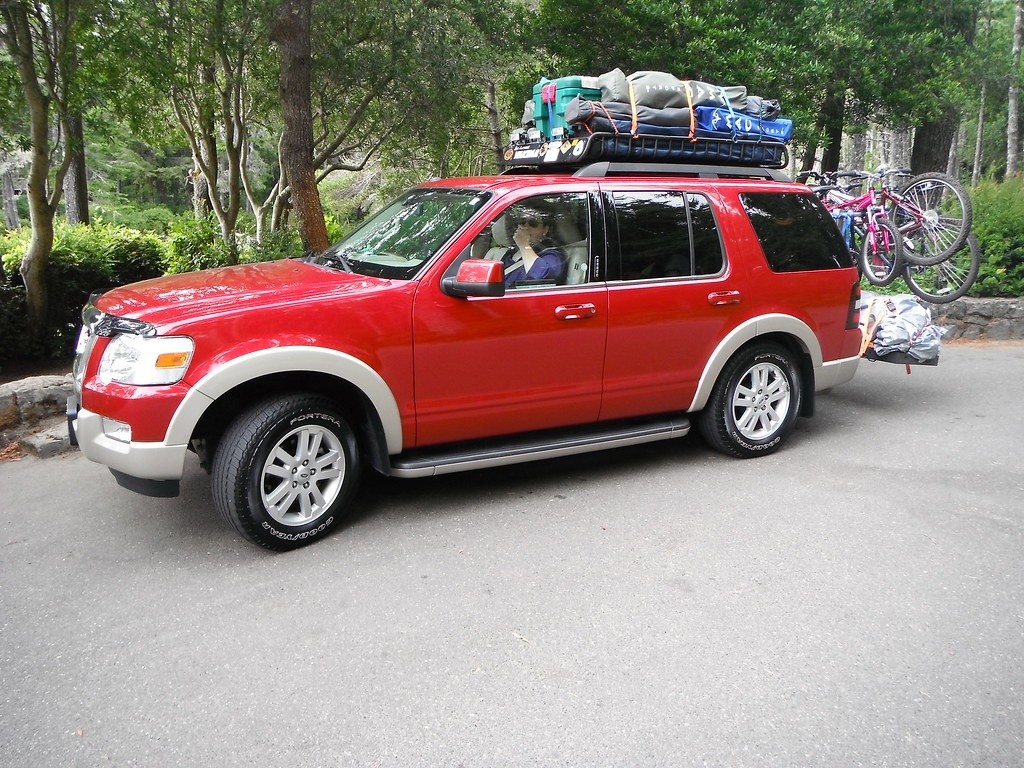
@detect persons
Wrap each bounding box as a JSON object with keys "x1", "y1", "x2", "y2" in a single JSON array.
[{"x1": 498, "y1": 205, "x2": 566, "y2": 291}]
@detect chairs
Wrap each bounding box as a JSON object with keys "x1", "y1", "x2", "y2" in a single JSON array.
[
  {"x1": 550, "y1": 218, "x2": 589, "y2": 285},
  {"x1": 483, "y1": 214, "x2": 515, "y2": 262}
]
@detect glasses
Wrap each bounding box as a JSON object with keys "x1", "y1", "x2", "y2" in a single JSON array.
[{"x1": 518, "y1": 217, "x2": 544, "y2": 228}]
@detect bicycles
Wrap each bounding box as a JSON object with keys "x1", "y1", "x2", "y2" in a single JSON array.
[{"x1": 794, "y1": 165, "x2": 980, "y2": 305}]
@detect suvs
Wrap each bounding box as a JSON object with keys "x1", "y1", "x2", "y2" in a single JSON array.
[{"x1": 65, "y1": 161, "x2": 863, "y2": 552}]
